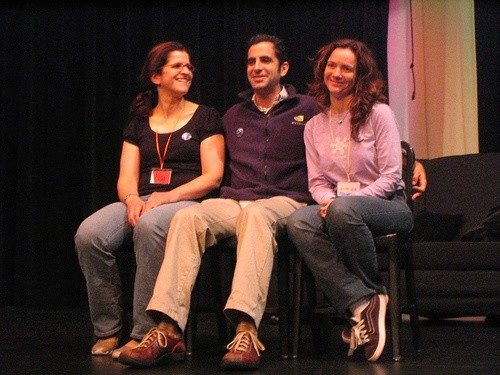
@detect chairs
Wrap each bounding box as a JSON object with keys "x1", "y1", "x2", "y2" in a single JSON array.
[{"x1": 291, "y1": 139, "x2": 422, "y2": 363}]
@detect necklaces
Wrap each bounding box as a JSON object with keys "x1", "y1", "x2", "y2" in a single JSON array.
[{"x1": 330, "y1": 109, "x2": 350, "y2": 124}]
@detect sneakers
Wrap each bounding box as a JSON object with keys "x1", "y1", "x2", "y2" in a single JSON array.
[
  {"x1": 342, "y1": 294, "x2": 389, "y2": 362},
  {"x1": 220, "y1": 330, "x2": 265, "y2": 368},
  {"x1": 118, "y1": 327, "x2": 185, "y2": 367}
]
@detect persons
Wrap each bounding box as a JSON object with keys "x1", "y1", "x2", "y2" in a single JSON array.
[
  {"x1": 75, "y1": 41, "x2": 225, "y2": 361},
  {"x1": 286, "y1": 39, "x2": 414, "y2": 362},
  {"x1": 117, "y1": 35, "x2": 428, "y2": 370}
]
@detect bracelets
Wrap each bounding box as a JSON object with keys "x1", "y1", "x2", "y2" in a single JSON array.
[{"x1": 123, "y1": 193, "x2": 138, "y2": 204}]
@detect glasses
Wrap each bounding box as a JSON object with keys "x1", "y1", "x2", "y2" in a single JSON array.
[{"x1": 161, "y1": 63, "x2": 195, "y2": 73}]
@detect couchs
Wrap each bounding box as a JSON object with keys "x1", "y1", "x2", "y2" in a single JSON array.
[{"x1": 375, "y1": 152, "x2": 500, "y2": 319}]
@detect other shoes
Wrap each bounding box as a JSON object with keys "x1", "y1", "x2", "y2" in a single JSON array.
[
  {"x1": 112, "y1": 340, "x2": 140, "y2": 360},
  {"x1": 91, "y1": 336, "x2": 119, "y2": 356}
]
